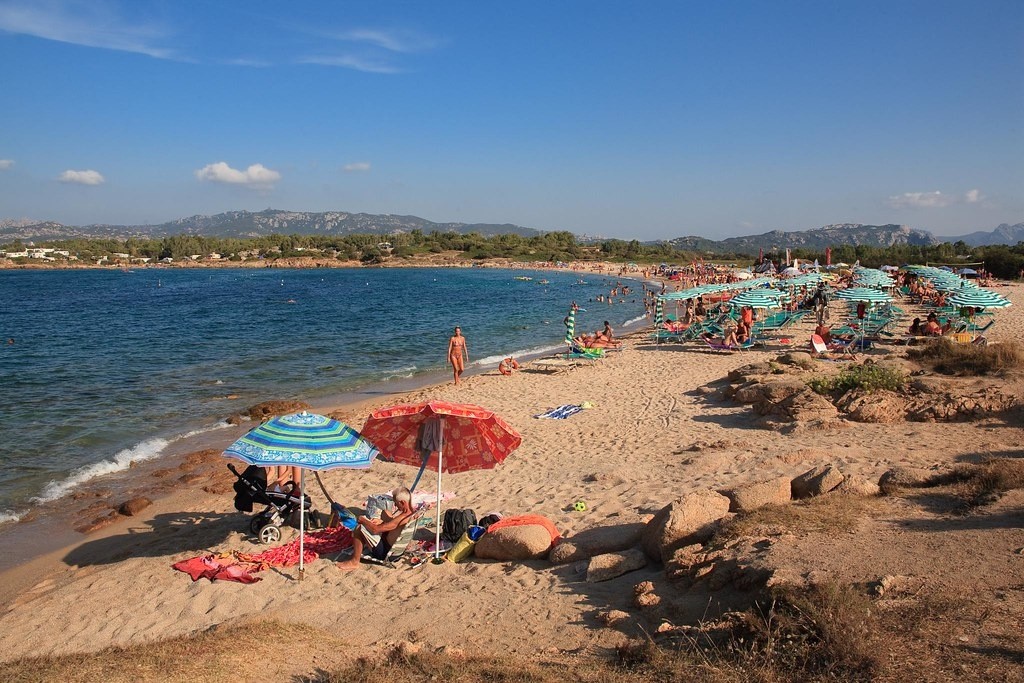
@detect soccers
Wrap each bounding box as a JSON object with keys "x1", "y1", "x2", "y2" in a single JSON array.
[{"x1": 575, "y1": 501, "x2": 586, "y2": 511}]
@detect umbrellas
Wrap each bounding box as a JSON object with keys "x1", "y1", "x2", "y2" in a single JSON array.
[
  {"x1": 358, "y1": 400, "x2": 520, "y2": 558},
  {"x1": 221, "y1": 411, "x2": 380, "y2": 570},
  {"x1": 565, "y1": 263, "x2": 1012, "y2": 355}
]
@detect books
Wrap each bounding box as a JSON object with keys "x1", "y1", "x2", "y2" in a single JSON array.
[{"x1": 360, "y1": 515, "x2": 381, "y2": 548}]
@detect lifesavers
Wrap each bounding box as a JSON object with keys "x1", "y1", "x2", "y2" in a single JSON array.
[{"x1": 499, "y1": 358, "x2": 518, "y2": 375}]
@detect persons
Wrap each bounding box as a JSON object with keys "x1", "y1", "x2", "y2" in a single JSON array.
[
  {"x1": 448, "y1": 326, "x2": 468, "y2": 386},
  {"x1": 266, "y1": 465, "x2": 301, "y2": 496},
  {"x1": 533, "y1": 261, "x2": 993, "y2": 348},
  {"x1": 334, "y1": 487, "x2": 418, "y2": 570}
]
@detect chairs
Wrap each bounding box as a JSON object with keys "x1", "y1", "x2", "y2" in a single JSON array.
[
  {"x1": 334, "y1": 502, "x2": 428, "y2": 569},
  {"x1": 532, "y1": 343, "x2": 626, "y2": 374},
  {"x1": 640, "y1": 282, "x2": 995, "y2": 359}
]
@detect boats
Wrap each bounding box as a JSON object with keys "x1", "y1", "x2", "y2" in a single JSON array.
[
  {"x1": 575, "y1": 282, "x2": 588, "y2": 285},
  {"x1": 514, "y1": 277, "x2": 533, "y2": 280},
  {"x1": 538, "y1": 281, "x2": 549, "y2": 284}
]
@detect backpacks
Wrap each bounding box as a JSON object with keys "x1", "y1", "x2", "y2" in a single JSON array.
[
  {"x1": 443, "y1": 509, "x2": 477, "y2": 543},
  {"x1": 233, "y1": 465, "x2": 267, "y2": 502}
]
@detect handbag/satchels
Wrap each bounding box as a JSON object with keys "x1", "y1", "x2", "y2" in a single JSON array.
[{"x1": 366, "y1": 494, "x2": 394, "y2": 519}]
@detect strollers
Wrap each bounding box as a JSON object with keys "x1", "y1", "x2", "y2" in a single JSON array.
[{"x1": 227, "y1": 462, "x2": 322, "y2": 545}]
